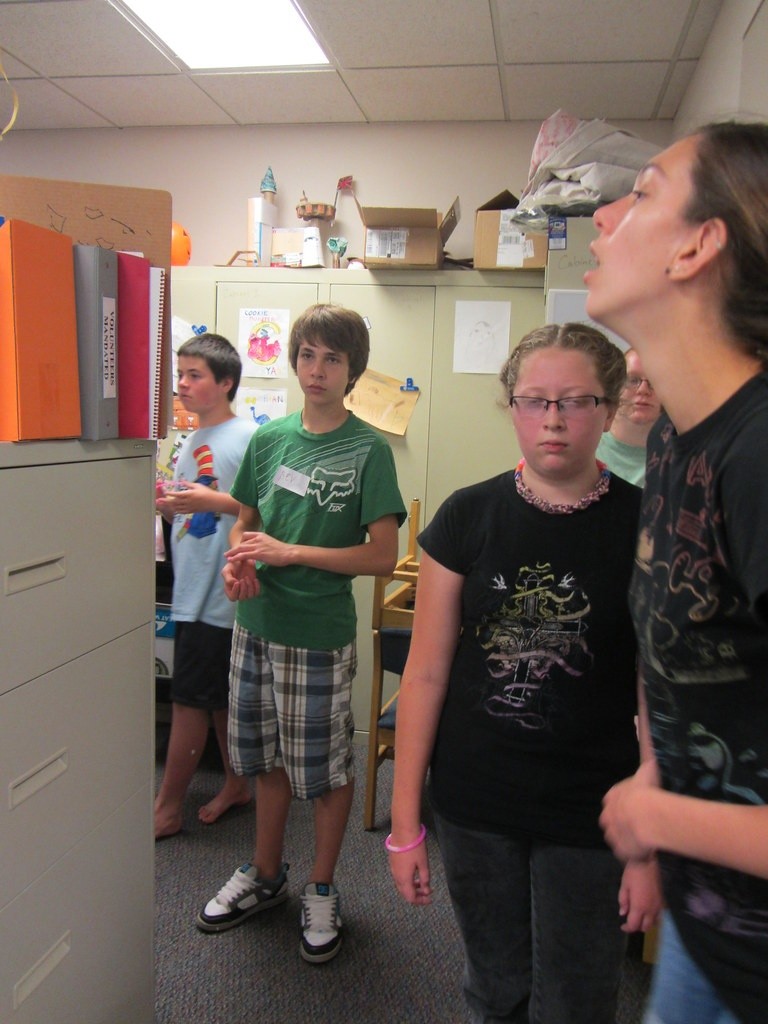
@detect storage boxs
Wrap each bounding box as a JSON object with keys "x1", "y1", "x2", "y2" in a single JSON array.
[
  {"x1": 349, "y1": 191, "x2": 461, "y2": 268},
  {"x1": 155, "y1": 606, "x2": 174, "y2": 678},
  {"x1": 473, "y1": 188, "x2": 549, "y2": 271}
]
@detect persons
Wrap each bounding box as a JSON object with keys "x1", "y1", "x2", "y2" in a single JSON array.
[
  {"x1": 595, "y1": 347, "x2": 664, "y2": 491},
  {"x1": 150, "y1": 333, "x2": 261, "y2": 841},
  {"x1": 196, "y1": 305, "x2": 408, "y2": 963},
  {"x1": 583, "y1": 119, "x2": 768, "y2": 1024},
  {"x1": 389, "y1": 322, "x2": 644, "y2": 1024}
]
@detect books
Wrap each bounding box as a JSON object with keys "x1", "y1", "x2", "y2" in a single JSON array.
[{"x1": 117, "y1": 251, "x2": 167, "y2": 441}]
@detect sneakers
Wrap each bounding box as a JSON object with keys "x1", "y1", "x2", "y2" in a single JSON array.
[
  {"x1": 196, "y1": 861, "x2": 291, "y2": 932},
  {"x1": 297, "y1": 881, "x2": 342, "y2": 962}
]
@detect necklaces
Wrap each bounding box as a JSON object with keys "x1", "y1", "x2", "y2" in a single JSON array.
[{"x1": 515, "y1": 458, "x2": 609, "y2": 515}]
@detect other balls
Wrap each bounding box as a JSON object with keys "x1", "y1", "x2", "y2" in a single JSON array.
[{"x1": 171, "y1": 222, "x2": 191, "y2": 266}]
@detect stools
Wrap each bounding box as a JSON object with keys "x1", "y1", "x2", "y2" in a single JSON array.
[{"x1": 372, "y1": 497, "x2": 421, "y2": 631}]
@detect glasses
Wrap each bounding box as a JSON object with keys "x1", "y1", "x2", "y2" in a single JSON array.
[
  {"x1": 623, "y1": 375, "x2": 655, "y2": 391},
  {"x1": 506, "y1": 389, "x2": 612, "y2": 417}
]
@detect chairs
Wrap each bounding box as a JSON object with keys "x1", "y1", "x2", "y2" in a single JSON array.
[{"x1": 363, "y1": 627, "x2": 411, "y2": 827}]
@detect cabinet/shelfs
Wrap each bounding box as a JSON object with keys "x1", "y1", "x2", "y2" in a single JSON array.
[
  {"x1": 0, "y1": 440, "x2": 159, "y2": 1024},
  {"x1": 170, "y1": 217, "x2": 630, "y2": 732}
]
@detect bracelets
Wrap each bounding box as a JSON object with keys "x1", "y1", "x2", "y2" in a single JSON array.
[{"x1": 385, "y1": 824, "x2": 427, "y2": 852}]
